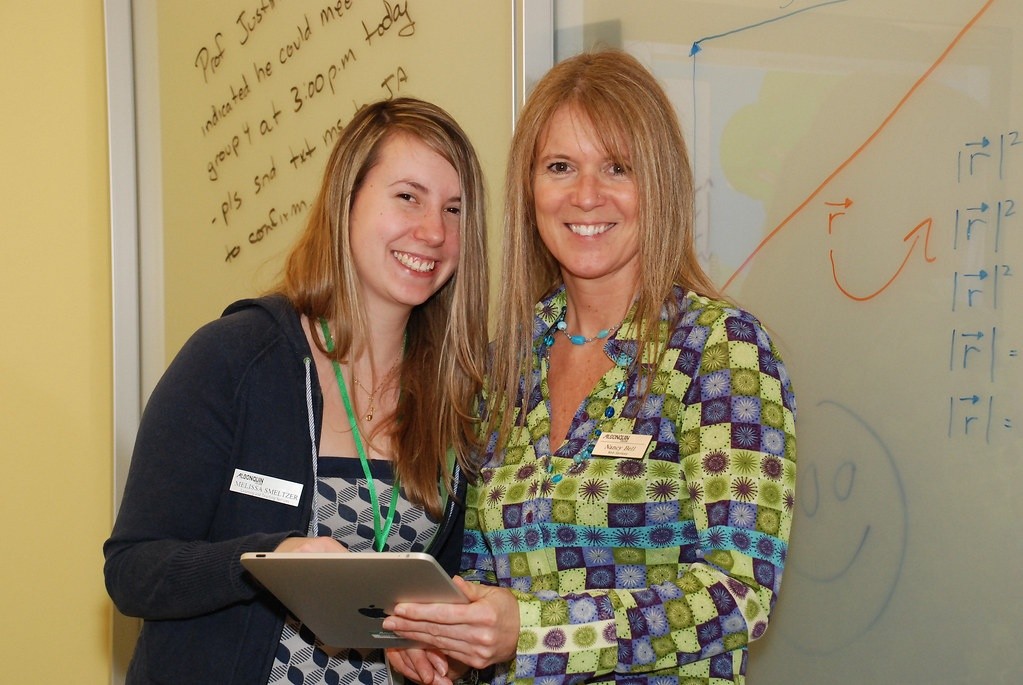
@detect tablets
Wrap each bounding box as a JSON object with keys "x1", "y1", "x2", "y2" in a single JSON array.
[{"x1": 241, "y1": 552, "x2": 473, "y2": 649}]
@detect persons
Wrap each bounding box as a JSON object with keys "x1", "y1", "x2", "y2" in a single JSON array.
[
  {"x1": 386, "y1": 48, "x2": 797, "y2": 685},
  {"x1": 103, "y1": 97, "x2": 491, "y2": 685}
]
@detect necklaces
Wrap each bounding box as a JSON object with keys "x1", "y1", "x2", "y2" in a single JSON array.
[
  {"x1": 331, "y1": 335, "x2": 404, "y2": 421},
  {"x1": 545, "y1": 308, "x2": 627, "y2": 482}
]
